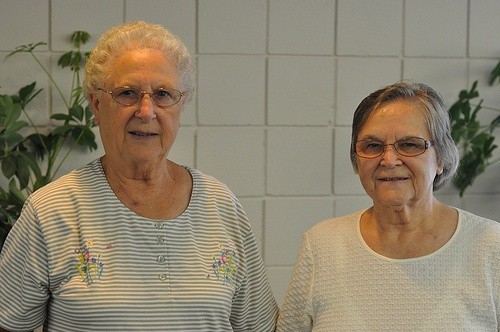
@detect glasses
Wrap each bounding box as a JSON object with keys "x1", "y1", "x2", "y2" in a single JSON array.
[
  {"x1": 355, "y1": 137, "x2": 434, "y2": 158},
  {"x1": 97, "y1": 85, "x2": 187, "y2": 107}
]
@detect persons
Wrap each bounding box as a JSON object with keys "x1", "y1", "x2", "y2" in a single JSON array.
[
  {"x1": 0, "y1": 21, "x2": 279, "y2": 332},
  {"x1": 274, "y1": 83, "x2": 499, "y2": 332}
]
